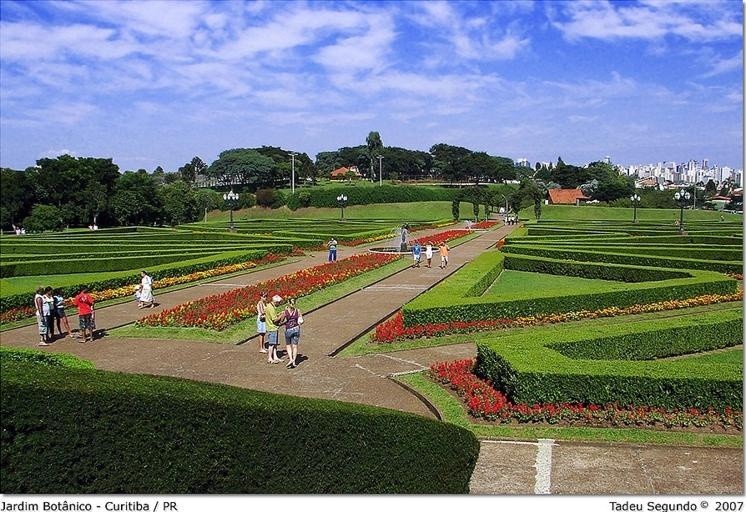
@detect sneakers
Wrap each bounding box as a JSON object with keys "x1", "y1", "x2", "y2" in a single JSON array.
[
  {"x1": 413, "y1": 265, "x2": 446, "y2": 269},
  {"x1": 258, "y1": 348, "x2": 297, "y2": 368}
]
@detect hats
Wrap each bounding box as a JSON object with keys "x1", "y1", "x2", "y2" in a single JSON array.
[
  {"x1": 259, "y1": 291, "x2": 267, "y2": 298},
  {"x1": 273, "y1": 295, "x2": 283, "y2": 302}
]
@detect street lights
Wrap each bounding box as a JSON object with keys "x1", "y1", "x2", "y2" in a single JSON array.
[
  {"x1": 376, "y1": 154, "x2": 385, "y2": 187},
  {"x1": 628, "y1": 193, "x2": 641, "y2": 222},
  {"x1": 336, "y1": 192, "x2": 348, "y2": 221},
  {"x1": 288, "y1": 150, "x2": 299, "y2": 195},
  {"x1": 222, "y1": 190, "x2": 239, "y2": 224},
  {"x1": 674, "y1": 187, "x2": 691, "y2": 233}
]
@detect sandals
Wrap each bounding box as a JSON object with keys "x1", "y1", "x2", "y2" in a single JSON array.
[{"x1": 37, "y1": 332, "x2": 93, "y2": 346}]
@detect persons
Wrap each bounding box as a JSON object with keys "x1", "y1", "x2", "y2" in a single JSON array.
[
  {"x1": 437, "y1": 241, "x2": 450, "y2": 269},
  {"x1": 504, "y1": 215, "x2": 515, "y2": 226},
  {"x1": 34, "y1": 285, "x2": 95, "y2": 346},
  {"x1": 139, "y1": 271, "x2": 156, "y2": 309},
  {"x1": 412, "y1": 242, "x2": 421, "y2": 268},
  {"x1": 14, "y1": 227, "x2": 21, "y2": 235},
  {"x1": 257, "y1": 292, "x2": 304, "y2": 368},
  {"x1": 134, "y1": 284, "x2": 143, "y2": 307},
  {"x1": 88, "y1": 224, "x2": 93, "y2": 231},
  {"x1": 467, "y1": 220, "x2": 472, "y2": 229},
  {"x1": 423, "y1": 240, "x2": 434, "y2": 268},
  {"x1": 327, "y1": 237, "x2": 338, "y2": 262},
  {"x1": 20, "y1": 228, "x2": 25, "y2": 234}
]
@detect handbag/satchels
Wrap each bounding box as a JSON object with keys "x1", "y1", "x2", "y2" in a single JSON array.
[
  {"x1": 272, "y1": 314, "x2": 287, "y2": 326},
  {"x1": 260, "y1": 314, "x2": 265, "y2": 322},
  {"x1": 296, "y1": 309, "x2": 303, "y2": 326}
]
